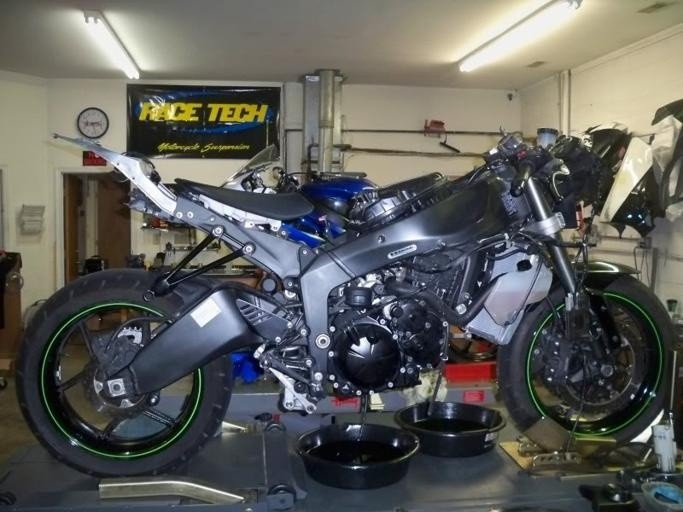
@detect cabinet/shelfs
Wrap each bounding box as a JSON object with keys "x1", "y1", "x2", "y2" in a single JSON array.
[{"x1": 139, "y1": 225, "x2": 219, "y2": 252}]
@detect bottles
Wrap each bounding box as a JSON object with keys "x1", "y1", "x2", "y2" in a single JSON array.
[{"x1": 162, "y1": 242, "x2": 175, "y2": 265}]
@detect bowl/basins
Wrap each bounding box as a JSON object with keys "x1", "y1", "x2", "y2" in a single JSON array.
[{"x1": 294, "y1": 401, "x2": 507, "y2": 490}]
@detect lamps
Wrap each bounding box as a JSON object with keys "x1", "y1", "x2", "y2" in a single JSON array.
[
  {"x1": 80, "y1": 7, "x2": 141, "y2": 80},
  {"x1": 457, "y1": 1, "x2": 582, "y2": 73}
]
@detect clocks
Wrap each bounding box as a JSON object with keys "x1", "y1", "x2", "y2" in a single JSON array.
[{"x1": 76, "y1": 107, "x2": 109, "y2": 139}]
